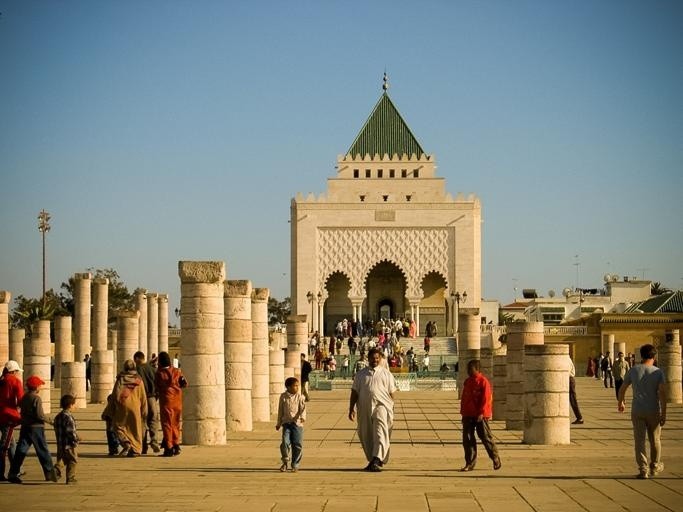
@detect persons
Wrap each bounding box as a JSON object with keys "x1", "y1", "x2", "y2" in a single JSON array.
[
  {"x1": 7, "y1": 376, "x2": 59, "y2": 483},
  {"x1": 0, "y1": 360, "x2": 25, "y2": 482},
  {"x1": 275, "y1": 378, "x2": 306, "y2": 473},
  {"x1": 300, "y1": 353, "x2": 313, "y2": 402},
  {"x1": 569, "y1": 357, "x2": 583, "y2": 424},
  {"x1": 52, "y1": 395, "x2": 81, "y2": 484},
  {"x1": 458, "y1": 360, "x2": 501, "y2": 472},
  {"x1": 616, "y1": 344, "x2": 667, "y2": 480},
  {"x1": 308, "y1": 314, "x2": 457, "y2": 381},
  {"x1": 82, "y1": 350, "x2": 188, "y2": 458},
  {"x1": 585, "y1": 351, "x2": 659, "y2": 404},
  {"x1": 348, "y1": 349, "x2": 396, "y2": 471}
]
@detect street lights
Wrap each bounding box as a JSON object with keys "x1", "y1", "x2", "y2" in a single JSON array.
[
  {"x1": 306, "y1": 290, "x2": 329, "y2": 336},
  {"x1": 451, "y1": 290, "x2": 467, "y2": 308},
  {"x1": 35, "y1": 209, "x2": 50, "y2": 305}
]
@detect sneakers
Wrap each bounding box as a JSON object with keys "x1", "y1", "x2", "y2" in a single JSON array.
[
  {"x1": 291, "y1": 466, "x2": 298, "y2": 472},
  {"x1": 640, "y1": 469, "x2": 649, "y2": 479},
  {"x1": 572, "y1": 419, "x2": 584, "y2": 423},
  {"x1": 494, "y1": 457, "x2": 501, "y2": 469},
  {"x1": 110, "y1": 440, "x2": 183, "y2": 457},
  {"x1": 605, "y1": 385, "x2": 608, "y2": 388},
  {"x1": 8, "y1": 476, "x2": 22, "y2": 484},
  {"x1": 304, "y1": 398, "x2": 309, "y2": 402},
  {"x1": 366, "y1": 462, "x2": 383, "y2": 472},
  {"x1": 461, "y1": 465, "x2": 473, "y2": 470},
  {"x1": 651, "y1": 462, "x2": 665, "y2": 475},
  {"x1": 67, "y1": 480, "x2": 77, "y2": 484},
  {"x1": 610, "y1": 385, "x2": 614, "y2": 388},
  {"x1": 279, "y1": 462, "x2": 288, "y2": 472},
  {"x1": 16, "y1": 471, "x2": 26, "y2": 476},
  {"x1": 45, "y1": 477, "x2": 58, "y2": 482},
  {"x1": 1, "y1": 477, "x2": 8, "y2": 482}
]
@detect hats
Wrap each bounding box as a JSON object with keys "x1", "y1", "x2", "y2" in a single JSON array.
[
  {"x1": 5, "y1": 360, "x2": 24, "y2": 372},
  {"x1": 26, "y1": 375, "x2": 45, "y2": 387}
]
